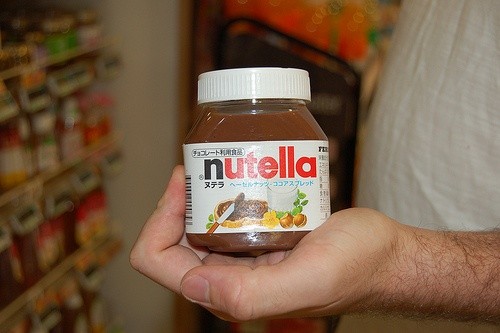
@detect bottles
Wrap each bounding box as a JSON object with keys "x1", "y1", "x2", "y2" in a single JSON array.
[{"x1": 180, "y1": 66, "x2": 332, "y2": 258}]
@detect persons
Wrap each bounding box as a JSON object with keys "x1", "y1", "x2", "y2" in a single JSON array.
[{"x1": 129, "y1": 0, "x2": 500, "y2": 333}]
